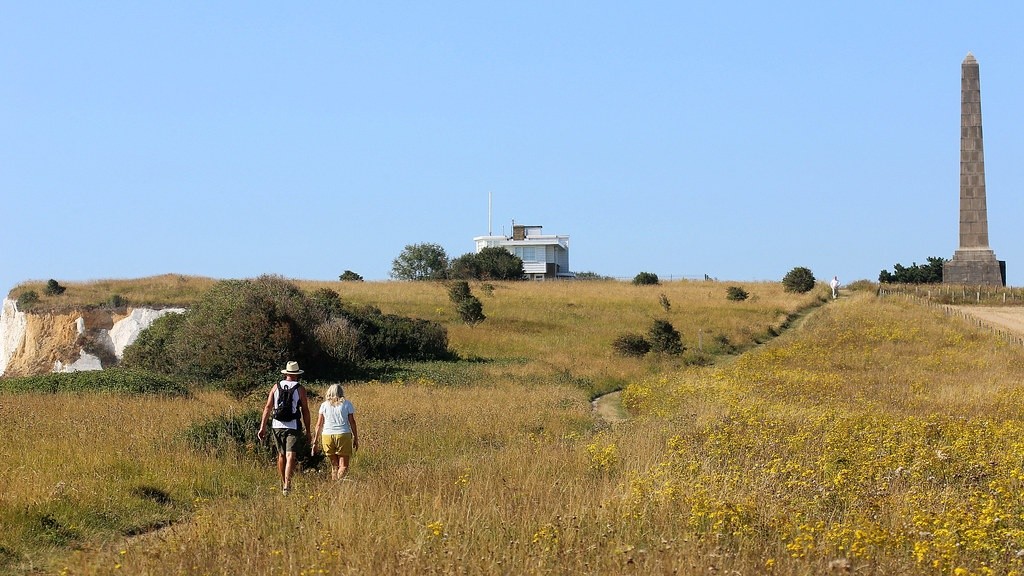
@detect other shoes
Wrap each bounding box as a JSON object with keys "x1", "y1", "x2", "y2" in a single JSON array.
[{"x1": 282, "y1": 487, "x2": 290, "y2": 494}]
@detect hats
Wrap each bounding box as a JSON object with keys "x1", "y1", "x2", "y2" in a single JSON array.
[{"x1": 281, "y1": 361, "x2": 305, "y2": 375}]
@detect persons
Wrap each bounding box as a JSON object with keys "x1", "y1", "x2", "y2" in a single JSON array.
[
  {"x1": 830, "y1": 275, "x2": 841, "y2": 301},
  {"x1": 311, "y1": 383, "x2": 359, "y2": 482},
  {"x1": 258, "y1": 361, "x2": 310, "y2": 498}
]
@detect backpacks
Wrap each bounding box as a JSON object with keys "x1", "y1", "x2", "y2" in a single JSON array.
[{"x1": 271, "y1": 378, "x2": 301, "y2": 421}]
